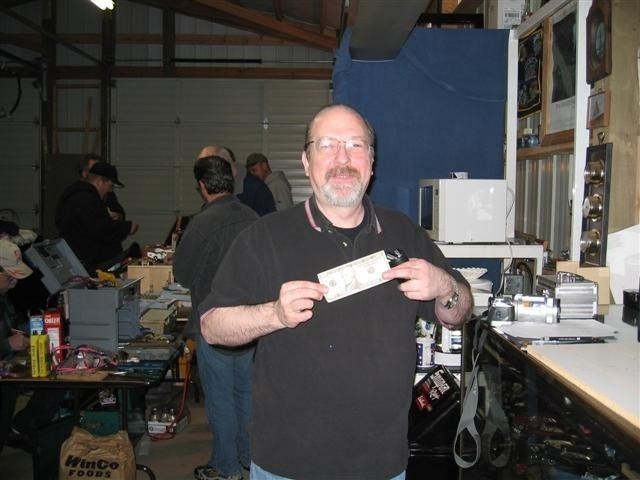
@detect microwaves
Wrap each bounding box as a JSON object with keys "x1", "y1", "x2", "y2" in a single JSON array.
[{"x1": 418, "y1": 178, "x2": 510, "y2": 244}]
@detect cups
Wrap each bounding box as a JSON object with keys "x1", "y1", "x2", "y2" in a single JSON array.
[
  {"x1": 578, "y1": 228, "x2": 601, "y2": 259},
  {"x1": 581, "y1": 193, "x2": 604, "y2": 221},
  {"x1": 622, "y1": 288, "x2": 639, "y2": 326},
  {"x1": 584, "y1": 159, "x2": 605, "y2": 182},
  {"x1": 523, "y1": 133, "x2": 538, "y2": 148}
]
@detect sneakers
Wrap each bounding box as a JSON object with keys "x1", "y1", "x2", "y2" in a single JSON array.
[{"x1": 193, "y1": 465, "x2": 243, "y2": 480}]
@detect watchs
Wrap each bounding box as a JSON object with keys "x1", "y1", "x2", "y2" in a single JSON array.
[{"x1": 440, "y1": 277, "x2": 459, "y2": 309}]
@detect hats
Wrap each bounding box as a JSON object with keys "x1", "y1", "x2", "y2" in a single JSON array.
[
  {"x1": 88, "y1": 162, "x2": 124, "y2": 188},
  {"x1": 0, "y1": 240, "x2": 34, "y2": 279},
  {"x1": 245, "y1": 153, "x2": 267, "y2": 167}
]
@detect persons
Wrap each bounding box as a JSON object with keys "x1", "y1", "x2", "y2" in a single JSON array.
[
  {"x1": 1, "y1": 152, "x2": 142, "y2": 478},
  {"x1": 171, "y1": 144, "x2": 294, "y2": 478},
  {"x1": 197, "y1": 103, "x2": 474, "y2": 479}
]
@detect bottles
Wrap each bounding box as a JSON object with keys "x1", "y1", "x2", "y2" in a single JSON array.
[
  {"x1": 521, "y1": 0, "x2": 532, "y2": 24},
  {"x1": 170, "y1": 215, "x2": 184, "y2": 252},
  {"x1": 104, "y1": 256, "x2": 132, "y2": 273},
  {"x1": 151, "y1": 407, "x2": 174, "y2": 423},
  {"x1": 27, "y1": 296, "x2": 63, "y2": 378}
]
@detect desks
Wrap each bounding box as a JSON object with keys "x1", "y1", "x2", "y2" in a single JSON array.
[
  {"x1": 7, "y1": 305, "x2": 193, "y2": 451},
  {"x1": 461, "y1": 310, "x2": 640, "y2": 480}
]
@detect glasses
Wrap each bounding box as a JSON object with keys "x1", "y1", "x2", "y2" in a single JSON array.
[{"x1": 305, "y1": 137, "x2": 374, "y2": 155}]
